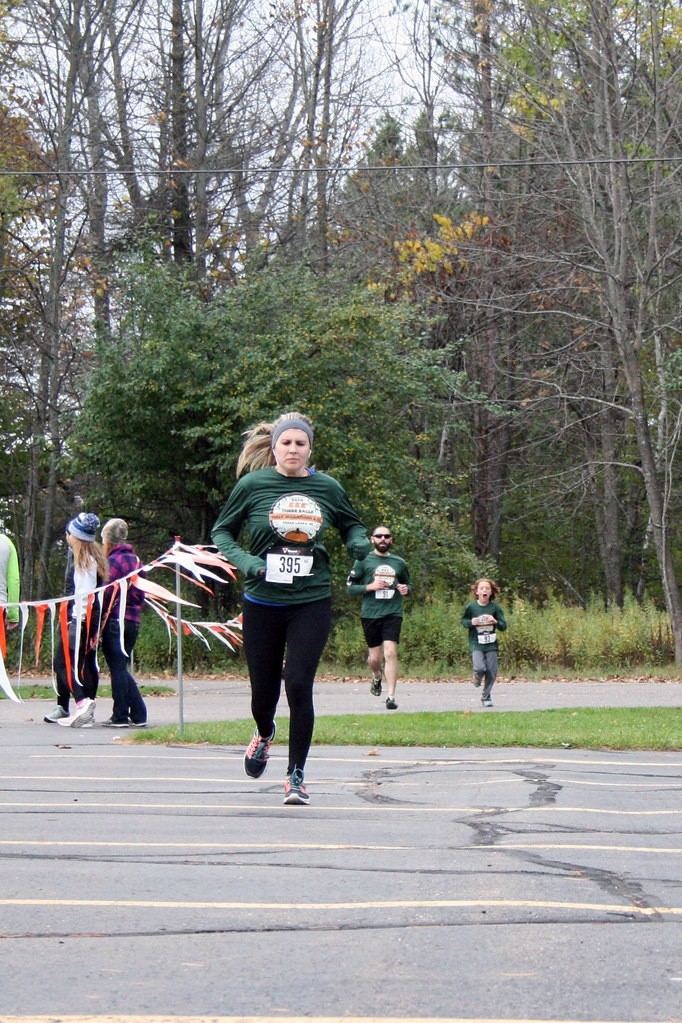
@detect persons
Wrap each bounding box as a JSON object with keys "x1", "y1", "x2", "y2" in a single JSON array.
[
  {"x1": 211, "y1": 413, "x2": 371, "y2": 804},
  {"x1": 100, "y1": 518, "x2": 148, "y2": 728},
  {"x1": 44, "y1": 512, "x2": 109, "y2": 726},
  {"x1": 460, "y1": 579, "x2": 507, "y2": 706},
  {"x1": 347, "y1": 526, "x2": 412, "y2": 709},
  {"x1": 0, "y1": 533, "x2": 20, "y2": 658}
]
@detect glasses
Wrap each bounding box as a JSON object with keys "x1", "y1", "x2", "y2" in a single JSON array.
[{"x1": 373, "y1": 533, "x2": 391, "y2": 539}]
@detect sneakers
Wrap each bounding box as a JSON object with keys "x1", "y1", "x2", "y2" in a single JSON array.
[
  {"x1": 58, "y1": 714, "x2": 96, "y2": 728},
  {"x1": 44, "y1": 705, "x2": 70, "y2": 723},
  {"x1": 385, "y1": 696, "x2": 398, "y2": 709},
  {"x1": 100, "y1": 718, "x2": 129, "y2": 728},
  {"x1": 128, "y1": 716, "x2": 148, "y2": 727},
  {"x1": 283, "y1": 763, "x2": 310, "y2": 805},
  {"x1": 242, "y1": 720, "x2": 276, "y2": 780},
  {"x1": 370, "y1": 668, "x2": 383, "y2": 696},
  {"x1": 70, "y1": 697, "x2": 96, "y2": 728}
]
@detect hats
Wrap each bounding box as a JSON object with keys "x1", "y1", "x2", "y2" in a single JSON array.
[
  {"x1": 65, "y1": 512, "x2": 100, "y2": 542},
  {"x1": 101, "y1": 518, "x2": 128, "y2": 545}
]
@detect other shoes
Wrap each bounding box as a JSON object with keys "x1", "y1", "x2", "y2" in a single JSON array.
[
  {"x1": 473, "y1": 673, "x2": 481, "y2": 687},
  {"x1": 482, "y1": 700, "x2": 493, "y2": 707}
]
